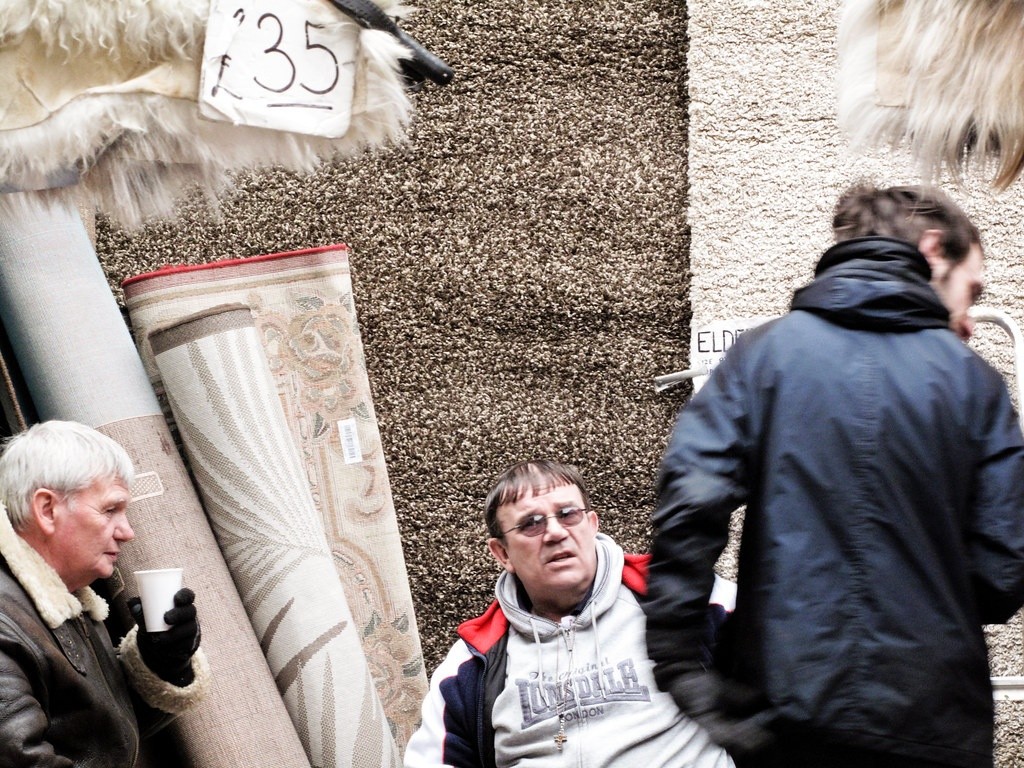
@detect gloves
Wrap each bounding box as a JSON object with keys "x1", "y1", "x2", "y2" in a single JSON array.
[{"x1": 127, "y1": 587, "x2": 201, "y2": 681}]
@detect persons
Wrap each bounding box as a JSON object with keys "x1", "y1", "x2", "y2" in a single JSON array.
[
  {"x1": 645, "y1": 186, "x2": 1024, "y2": 768},
  {"x1": 399, "y1": 459, "x2": 738, "y2": 768},
  {"x1": 0, "y1": 420, "x2": 209, "y2": 768}
]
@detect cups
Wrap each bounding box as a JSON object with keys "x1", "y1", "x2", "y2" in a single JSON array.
[{"x1": 133, "y1": 567, "x2": 184, "y2": 632}]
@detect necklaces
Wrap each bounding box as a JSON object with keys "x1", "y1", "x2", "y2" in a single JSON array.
[{"x1": 552, "y1": 620, "x2": 575, "y2": 750}]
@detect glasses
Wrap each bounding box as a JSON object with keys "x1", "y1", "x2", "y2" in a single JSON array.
[{"x1": 494, "y1": 506, "x2": 593, "y2": 537}]
